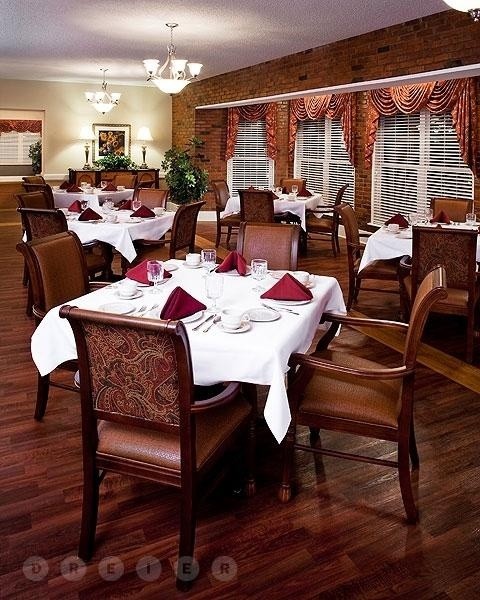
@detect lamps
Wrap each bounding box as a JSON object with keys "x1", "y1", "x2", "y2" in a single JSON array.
[
  {"x1": 83, "y1": 70, "x2": 121, "y2": 115},
  {"x1": 76, "y1": 126, "x2": 98, "y2": 170},
  {"x1": 135, "y1": 126, "x2": 154, "y2": 169},
  {"x1": 142, "y1": 21, "x2": 203, "y2": 96}
]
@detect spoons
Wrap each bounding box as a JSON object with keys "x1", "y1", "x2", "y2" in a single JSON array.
[{"x1": 204, "y1": 315, "x2": 222, "y2": 333}]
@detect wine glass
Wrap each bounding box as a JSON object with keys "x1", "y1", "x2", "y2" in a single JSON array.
[
  {"x1": 81, "y1": 200, "x2": 89, "y2": 214},
  {"x1": 407, "y1": 213, "x2": 418, "y2": 230},
  {"x1": 205, "y1": 276, "x2": 224, "y2": 313},
  {"x1": 276, "y1": 187, "x2": 282, "y2": 198},
  {"x1": 201, "y1": 248, "x2": 216, "y2": 279},
  {"x1": 101, "y1": 181, "x2": 107, "y2": 189},
  {"x1": 258, "y1": 186, "x2": 264, "y2": 190},
  {"x1": 251, "y1": 259, "x2": 267, "y2": 293},
  {"x1": 81, "y1": 182, "x2": 87, "y2": 196},
  {"x1": 147, "y1": 260, "x2": 165, "y2": 294},
  {"x1": 292, "y1": 185, "x2": 298, "y2": 195},
  {"x1": 133, "y1": 201, "x2": 142, "y2": 211},
  {"x1": 424, "y1": 208, "x2": 433, "y2": 225},
  {"x1": 466, "y1": 213, "x2": 476, "y2": 229}
]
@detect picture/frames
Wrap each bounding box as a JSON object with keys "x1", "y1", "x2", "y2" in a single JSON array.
[{"x1": 92, "y1": 124, "x2": 131, "y2": 163}]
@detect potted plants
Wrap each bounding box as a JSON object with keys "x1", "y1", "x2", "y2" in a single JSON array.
[{"x1": 27, "y1": 140, "x2": 42, "y2": 177}]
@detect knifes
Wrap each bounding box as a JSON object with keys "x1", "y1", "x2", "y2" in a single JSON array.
[
  {"x1": 108, "y1": 285, "x2": 150, "y2": 287},
  {"x1": 192, "y1": 314, "x2": 216, "y2": 330},
  {"x1": 210, "y1": 264, "x2": 221, "y2": 273}
]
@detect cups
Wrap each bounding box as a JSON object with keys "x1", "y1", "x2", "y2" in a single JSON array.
[
  {"x1": 287, "y1": 193, "x2": 296, "y2": 200},
  {"x1": 118, "y1": 210, "x2": 130, "y2": 221},
  {"x1": 87, "y1": 184, "x2": 91, "y2": 189},
  {"x1": 117, "y1": 186, "x2": 123, "y2": 190},
  {"x1": 292, "y1": 271, "x2": 315, "y2": 286},
  {"x1": 221, "y1": 308, "x2": 250, "y2": 330},
  {"x1": 186, "y1": 254, "x2": 201, "y2": 266},
  {"x1": 63, "y1": 209, "x2": 68, "y2": 214},
  {"x1": 119, "y1": 280, "x2": 137, "y2": 297},
  {"x1": 388, "y1": 224, "x2": 399, "y2": 232},
  {"x1": 154, "y1": 208, "x2": 163, "y2": 215}
]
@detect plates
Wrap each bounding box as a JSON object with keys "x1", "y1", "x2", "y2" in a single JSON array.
[
  {"x1": 244, "y1": 308, "x2": 280, "y2": 321},
  {"x1": 183, "y1": 261, "x2": 201, "y2": 269},
  {"x1": 164, "y1": 263, "x2": 179, "y2": 272},
  {"x1": 218, "y1": 266, "x2": 253, "y2": 276},
  {"x1": 271, "y1": 299, "x2": 310, "y2": 305},
  {"x1": 77, "y1": 219, "x2": 92, "y2": 223},
  {"x1": 114, "y1": 290, "x2": 144, "y2": 300},
  {"x1": 179, "y1": 309, "x2": 202, "y2": 323},
  {"x1": 137, "y1": 278, "x2": 171, "y2": 289},
  {"x1": 100, "y1": 301, "x2": 136, "y2": 315},
  {"x1": 221, "y1": 322, "x2": 251, "y2": 334}
]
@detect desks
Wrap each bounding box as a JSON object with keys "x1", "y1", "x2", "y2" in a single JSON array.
[{"x1": 69, "y1": 168, "x2": 160, "y2": 190}]
